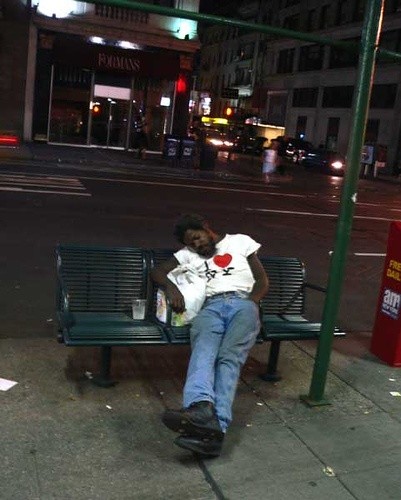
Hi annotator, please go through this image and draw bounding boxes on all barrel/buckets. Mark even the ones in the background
[155,286,189,326]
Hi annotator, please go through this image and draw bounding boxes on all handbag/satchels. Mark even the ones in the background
[157,262,207,326]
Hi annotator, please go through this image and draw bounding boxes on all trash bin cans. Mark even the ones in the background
[199,143,218,169]
[162,135,196,162]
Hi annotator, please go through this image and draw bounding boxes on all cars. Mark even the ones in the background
[195,127,346,174]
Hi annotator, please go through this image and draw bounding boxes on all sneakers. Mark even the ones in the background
[163,401,222,438]
[174,433,222,456]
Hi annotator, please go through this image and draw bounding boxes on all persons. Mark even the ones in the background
[262,143,277,184]
[151,213,269,458]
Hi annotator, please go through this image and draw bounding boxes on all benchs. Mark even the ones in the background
[47,245,346,388]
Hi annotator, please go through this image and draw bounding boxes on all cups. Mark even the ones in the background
[132,299,146,320]
